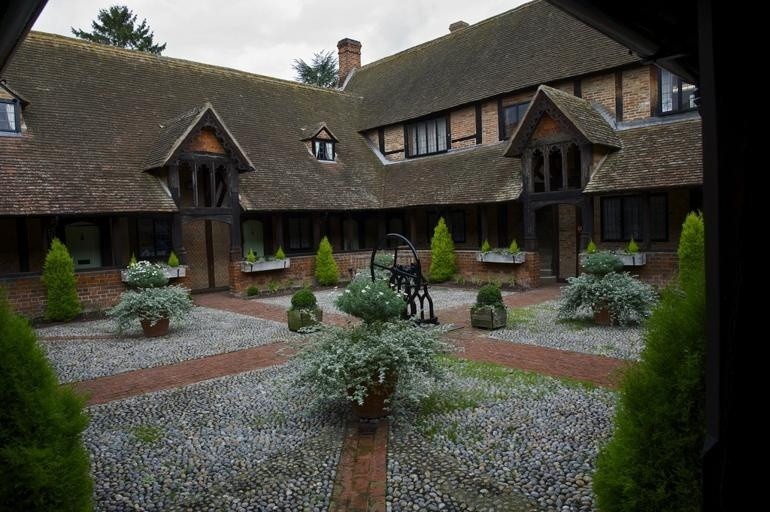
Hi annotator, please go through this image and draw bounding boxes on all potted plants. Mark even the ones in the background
[122,252,187,281]
[470,284,507,329]
[475,239,525,263]
[106,285,195,337]
[240,247,290,272]
[286,289,323,332]
[292,321,466,418]
[558,273,657,327]
[580,239,647,266]
